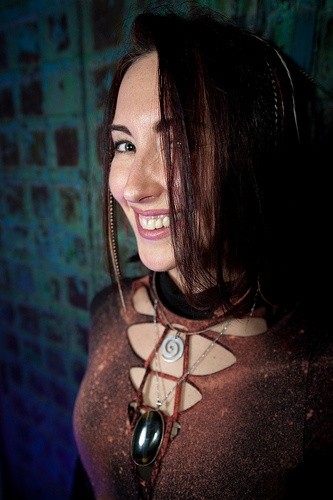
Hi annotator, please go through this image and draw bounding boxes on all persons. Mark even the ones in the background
[69,11,332,500]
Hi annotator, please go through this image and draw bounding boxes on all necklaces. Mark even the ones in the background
[129,301,237,468]
[148,271,259,365]
